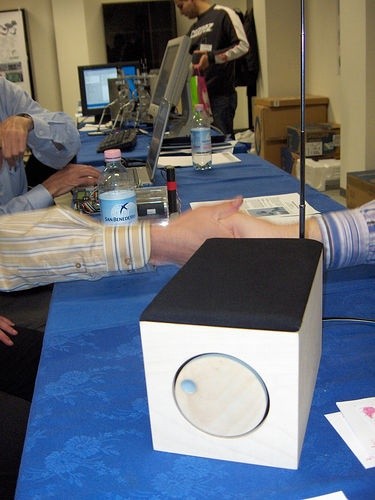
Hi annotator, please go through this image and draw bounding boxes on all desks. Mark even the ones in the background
[14,123,375,500]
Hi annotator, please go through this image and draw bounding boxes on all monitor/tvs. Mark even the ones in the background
[147,35,194,137]
[77,62,141,116]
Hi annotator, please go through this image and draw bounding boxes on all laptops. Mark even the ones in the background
[78,97,171,192]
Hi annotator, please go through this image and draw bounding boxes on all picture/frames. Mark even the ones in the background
[0,8,35,102]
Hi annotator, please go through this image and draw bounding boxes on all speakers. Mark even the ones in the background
[139,239,326,470]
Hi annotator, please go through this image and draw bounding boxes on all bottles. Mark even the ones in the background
[191,104,212,170]
[98,149,138,225]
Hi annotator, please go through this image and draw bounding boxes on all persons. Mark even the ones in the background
[0,196,244,483]
[0,77,101,333]
[220,199,375,271]
[174,0,250,140]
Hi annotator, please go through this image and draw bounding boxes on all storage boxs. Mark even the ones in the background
[138,234,325,471]
[285,124,344,160]
[253,94,329,107]
[295,160,347,210]
[252,103,327,167]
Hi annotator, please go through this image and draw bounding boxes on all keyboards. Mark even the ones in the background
[97,128,138,152]
